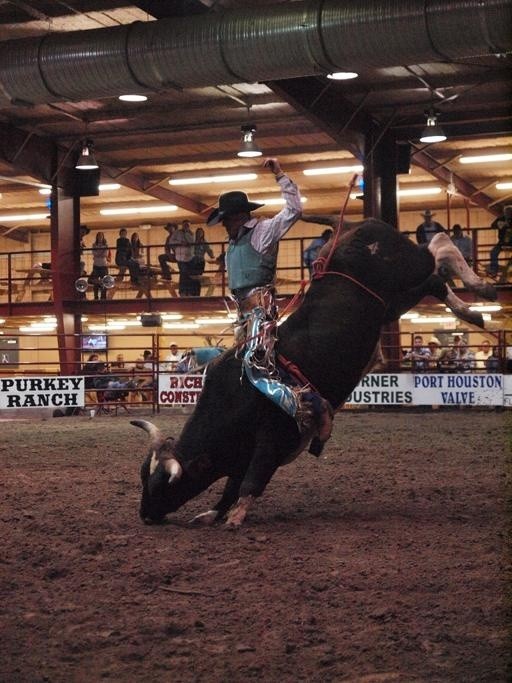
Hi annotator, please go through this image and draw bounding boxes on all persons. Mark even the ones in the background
[305,229,333,279]
[82,342,196,401]
[451,223,473,268]
[416,209,445,241]
[203,156,335,445]
[131,232,145,280]
[485,206,511,277]
[158,220,214,298]
[79,225,90,300]
[92,232,109,300]
[401,336,510,376]
[115,229,141,286]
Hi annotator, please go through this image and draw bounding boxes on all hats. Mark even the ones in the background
[421,210,436,217]
[207,191,265,227]
[428,337,441,346]
[164,223,178,230]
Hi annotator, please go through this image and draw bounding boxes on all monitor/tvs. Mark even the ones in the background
[81,332,107,352]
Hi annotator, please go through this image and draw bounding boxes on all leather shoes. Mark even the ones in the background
[318,397,332,441]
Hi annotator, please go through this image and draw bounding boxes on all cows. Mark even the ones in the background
[129,213,498,532]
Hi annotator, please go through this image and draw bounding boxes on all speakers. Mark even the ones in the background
[65,166,100,197]
[393,142,411,173]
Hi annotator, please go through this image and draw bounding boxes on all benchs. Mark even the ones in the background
[0,262,228,303]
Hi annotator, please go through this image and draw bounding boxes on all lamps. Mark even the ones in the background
[73,108,99,172]
[236,97,261,160]
[416,84,449,144]
